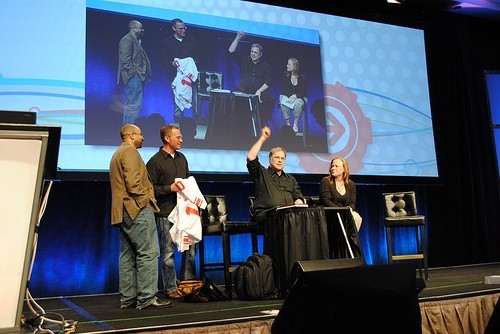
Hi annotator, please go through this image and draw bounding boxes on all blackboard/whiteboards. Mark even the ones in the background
[0,129,49,333]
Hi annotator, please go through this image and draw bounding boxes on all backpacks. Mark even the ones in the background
[233,253,275,301]
[178,116,197,148]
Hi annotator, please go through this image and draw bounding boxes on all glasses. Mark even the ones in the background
[129,132,142,136]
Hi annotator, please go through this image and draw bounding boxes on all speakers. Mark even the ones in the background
[286,258,363,297]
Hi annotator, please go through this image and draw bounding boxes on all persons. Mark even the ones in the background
[116,20,153,124]
[316,156,363,232]
[160,18,200,123]
[277,57,306,133]
[109,124,171,311]
[228,30,275,128]
[145,125,190,299]
[246,126,307,254]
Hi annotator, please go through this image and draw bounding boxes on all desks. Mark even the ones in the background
[204,89,262,145]
[266,207,362,299]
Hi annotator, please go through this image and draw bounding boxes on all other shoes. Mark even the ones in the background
[293,126,298,132]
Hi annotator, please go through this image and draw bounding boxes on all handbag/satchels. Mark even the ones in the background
[168,277,228,303]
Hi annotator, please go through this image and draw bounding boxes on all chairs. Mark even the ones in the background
[193,72,223,118]
[248,195,320,255]
[199,195,258,300]
[381,191,428,280]
[277,102,309,147]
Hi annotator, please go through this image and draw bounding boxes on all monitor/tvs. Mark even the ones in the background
[0,111,37,125]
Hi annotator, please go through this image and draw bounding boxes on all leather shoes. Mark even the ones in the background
[127,302,137,310]
[142,298,173,310]
